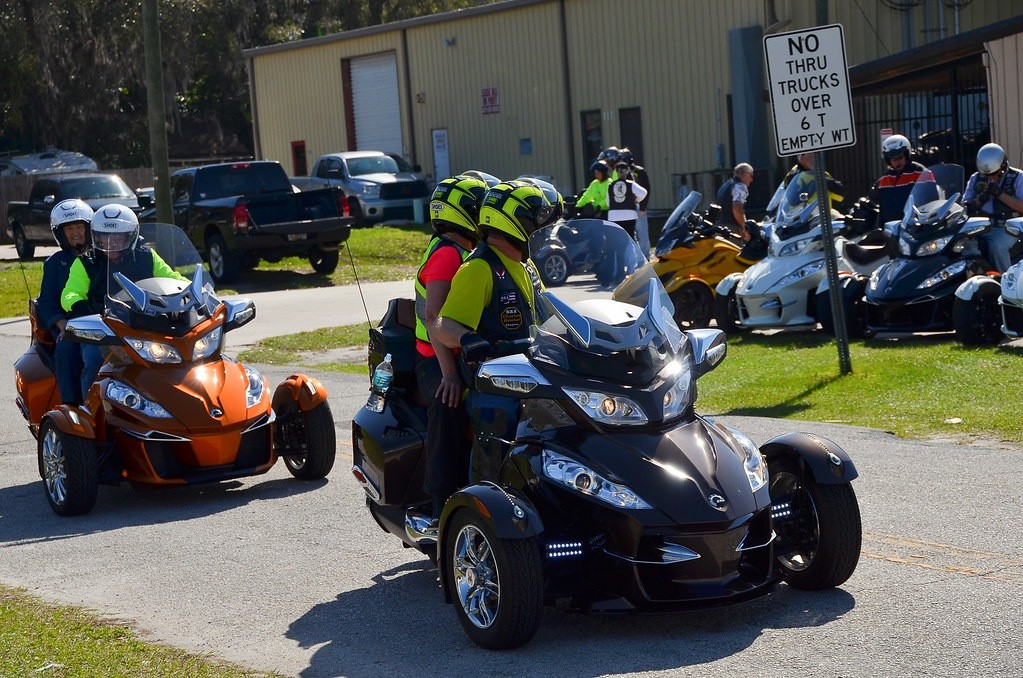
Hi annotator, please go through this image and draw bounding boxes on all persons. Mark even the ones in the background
[571,145,651,289]
[962,141,1023,274]
[873,134,940,231]
[409,166,566,524]
[714,161,753,243]
[783,151,844,211]
[35,199,207,414]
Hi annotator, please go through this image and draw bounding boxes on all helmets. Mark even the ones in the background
[92,204,139,258]
[597,147,620,164]
[49,199,94,252]
[590,160,612,183]
[431,170,502,250]
[479,177,564,260]
[976,144,1005,174]
[884,135,911,172]
[616,148,634,166]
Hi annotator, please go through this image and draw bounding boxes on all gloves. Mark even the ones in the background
[461,332,494,365]
[978,183,1002,202]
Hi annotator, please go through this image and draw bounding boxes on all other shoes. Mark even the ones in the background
[596,262,647,288]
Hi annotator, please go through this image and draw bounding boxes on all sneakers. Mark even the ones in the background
[404,506,440,544]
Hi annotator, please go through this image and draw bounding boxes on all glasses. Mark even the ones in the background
[892,156,905,163]
[985,170,999,176]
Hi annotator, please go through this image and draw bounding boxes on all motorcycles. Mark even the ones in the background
[527,187,609,287]
[610,164,1023,348]
[352,214,863,654]
[15,220,335,517]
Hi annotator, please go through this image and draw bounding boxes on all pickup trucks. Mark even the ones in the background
[289,149,433,229]
[6,170,146,261]
[137,161,353,286]
